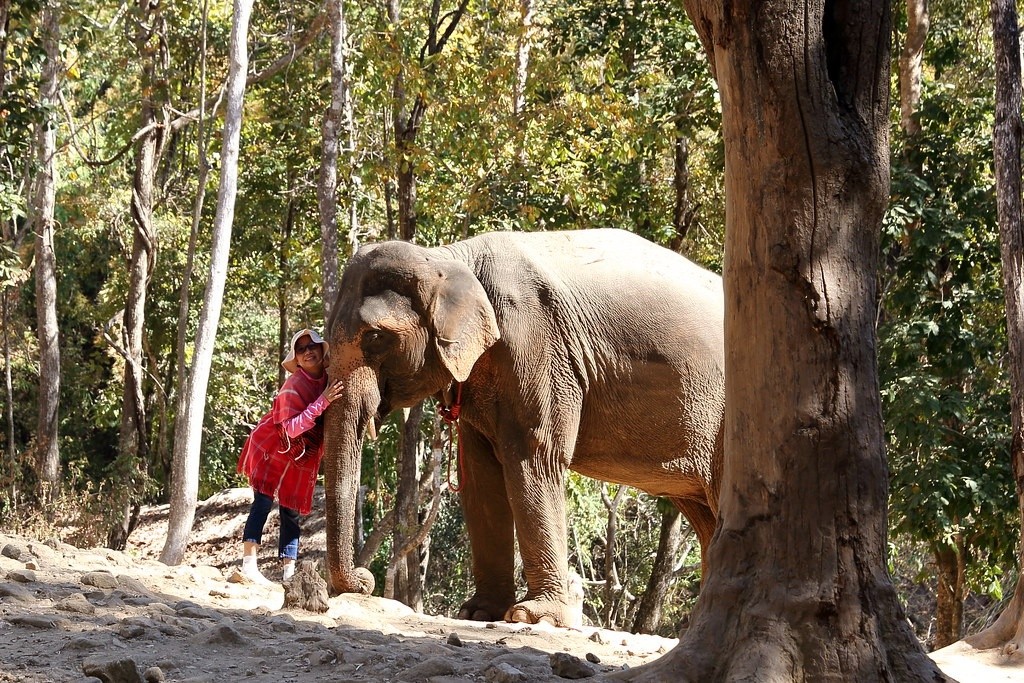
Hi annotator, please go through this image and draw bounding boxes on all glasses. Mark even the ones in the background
[295,340,318,354]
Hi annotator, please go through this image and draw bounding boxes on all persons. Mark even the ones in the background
[235,329,344,584]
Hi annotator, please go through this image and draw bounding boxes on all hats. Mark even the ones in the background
[281,328,331,373]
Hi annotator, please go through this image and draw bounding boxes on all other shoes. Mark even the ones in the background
[241,567,274,587]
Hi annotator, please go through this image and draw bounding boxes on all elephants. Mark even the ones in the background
[322,228,726,635]
